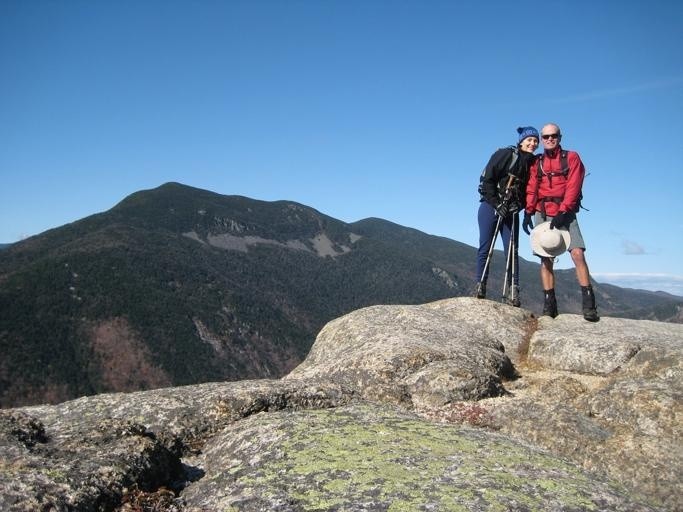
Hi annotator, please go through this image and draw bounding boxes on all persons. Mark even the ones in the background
[478,127,543,307]
[523,124,597,322]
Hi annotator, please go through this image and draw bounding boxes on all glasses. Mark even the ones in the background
[542,134,559,140]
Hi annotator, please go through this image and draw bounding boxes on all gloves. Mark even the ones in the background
[509,201,522,214]
[496,203,509,218]
[549,211,574,230]
[522,211,534,236]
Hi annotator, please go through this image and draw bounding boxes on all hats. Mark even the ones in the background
[528,221,571,259]
[516,126,540,146]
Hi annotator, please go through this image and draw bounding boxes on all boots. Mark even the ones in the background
[538,287,560,319]
[475,281,487,299]
[507,285,521,308]
[580,283,600,322]
